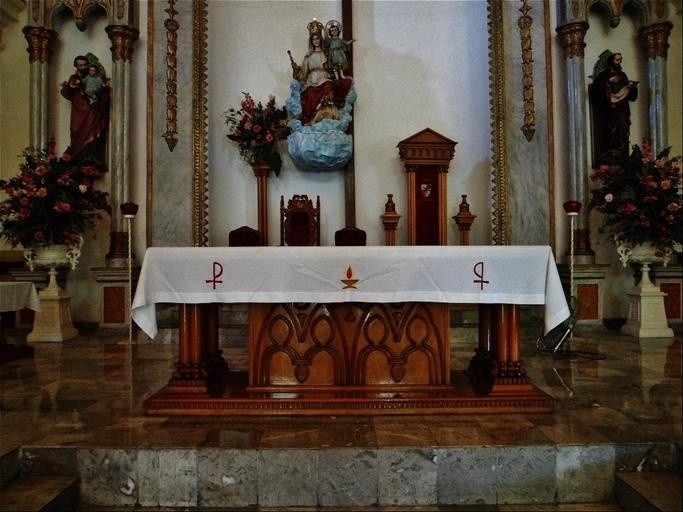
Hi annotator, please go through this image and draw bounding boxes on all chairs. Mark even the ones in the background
[281,195,320,246]
[334,225,366,246]
[229,226,261,246]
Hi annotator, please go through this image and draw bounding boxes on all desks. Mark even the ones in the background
[132,246,569,413]
[1,275,43,328]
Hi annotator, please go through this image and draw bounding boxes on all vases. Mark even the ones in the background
[616,238,673,267]
[24,243,78,268]
[254,152,265,161]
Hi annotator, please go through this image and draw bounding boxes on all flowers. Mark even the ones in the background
[223,91,291,178]
[587,137,683,245]
[0,132,112,245]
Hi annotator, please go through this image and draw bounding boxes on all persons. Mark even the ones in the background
[592,51,638,159]
[75,65,106,105]
[322,18,358,79]
[61,54,109,168]
[289,34,337,129]
